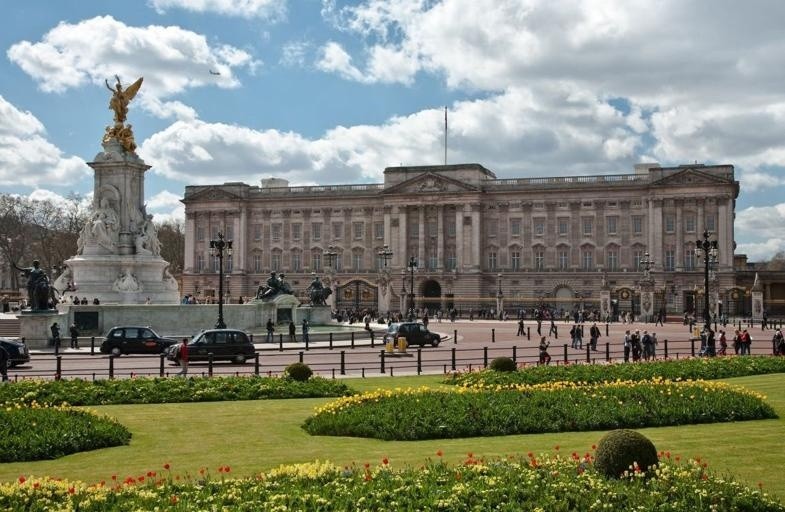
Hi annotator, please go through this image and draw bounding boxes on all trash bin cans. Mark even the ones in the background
[386,337,394,353]
[398,337,406,352]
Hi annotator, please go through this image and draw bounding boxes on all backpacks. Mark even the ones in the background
[166,342,184,361]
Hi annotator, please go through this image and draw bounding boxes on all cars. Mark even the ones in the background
[380,321,442,348]
[164,328,256,367]
[97,323,177,358]
[1,336,34,377]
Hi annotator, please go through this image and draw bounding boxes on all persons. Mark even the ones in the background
[181,294,214,304]
[516,316,526,336]
[539,336,552,366]
[0,294,8,312]
[105,78,130,126]
[116,123,137,152]
[301,319,310,342]
[10,257,46,309]
[17,299,27,310]
[289,320,298,342]
[69,323,81,350]
[682,309,785,356]
[175,337,190,378]
[277,273,294,294]
[243,295,249,304]
[203,334,213,344]
[264,319,274,343]
[623,329,659,364]
[655,308,664,327]
[88,195,120,254]
[144,296,152,305]
[238,296,243,304]
[536,317,543,336]
[476,305,635,324]
[50,322,61,348]
[548,319,559,337]
[570,325,578,348]
[56,280,100,305]
[306,275,324,304]
[330,307,476,338]
[89,212,114,238]
[143,214,161,257]
[10,303,19,313]
[574,324,585,350]
[254,270,281,299]
[590,322,602,351]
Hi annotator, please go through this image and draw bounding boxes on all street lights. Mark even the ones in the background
[206,231,235,329]
[497,271,503,296]
[378,242,395,267]
[694,227,721,357]
[406,255,419,316]
[323,243,338,267]
[47,265,57,283]
[639,252,656,282]
[310,270,316,281]
[701,254,720,273]
[223,272,232,304]
